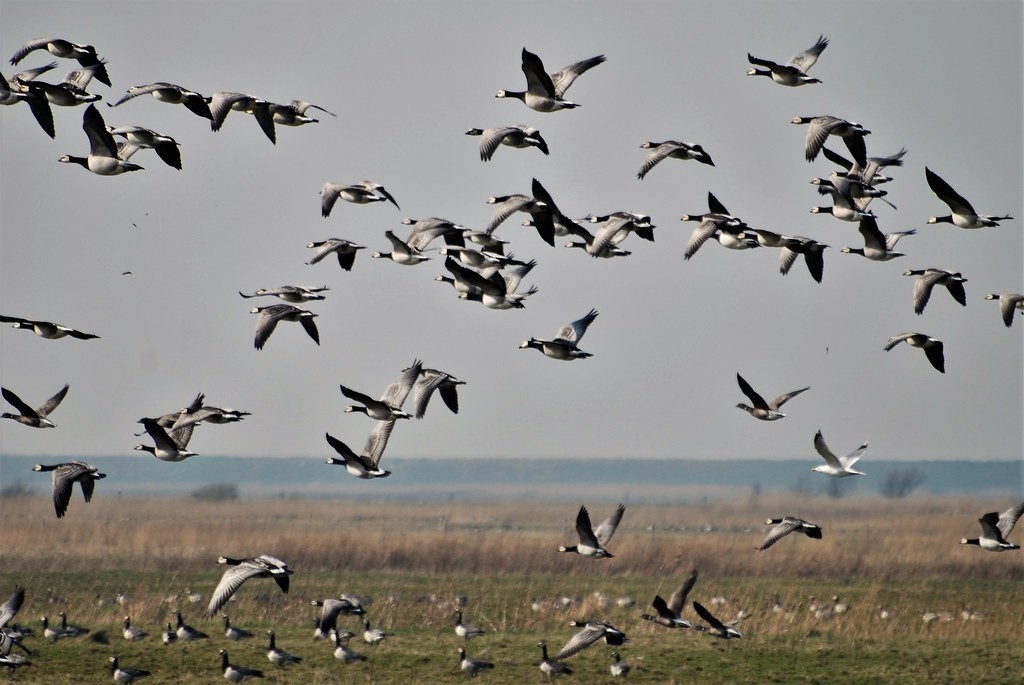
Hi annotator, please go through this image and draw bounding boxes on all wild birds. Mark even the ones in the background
[639,570,697,628]
[692,600,752,638]
[553,620,629,662]
[810,429,869,477]
[752,517,822,551]
[958,502,1024,551]
[455,608,486,640]
[537,642,574,684]
[528,593,987,628]
[132,393,252,462]
[0,315,101,340]
[555,504,627,559]
[0,33,1024,437]
[1,385,69,428]
[735,373,811,421]
[0,556,395,685]
[609,653,628,677]
[32,462,107,519]
[457,648,496,677]
[325,419,394,478]
